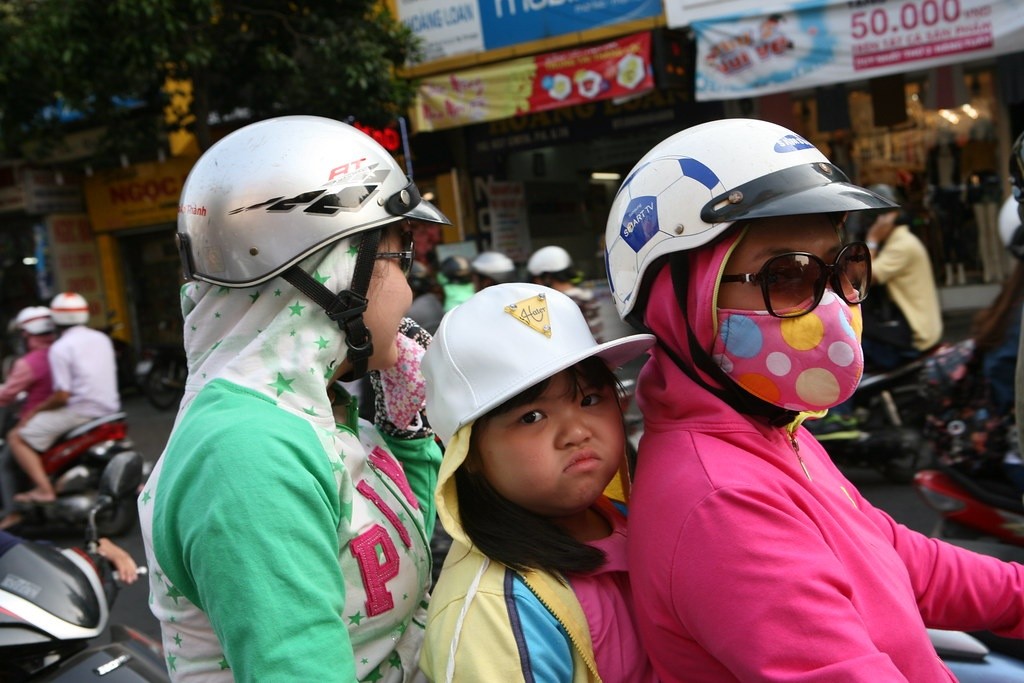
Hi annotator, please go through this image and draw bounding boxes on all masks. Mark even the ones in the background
[711,288,864,410]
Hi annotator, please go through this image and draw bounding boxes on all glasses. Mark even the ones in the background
[720,242,872,318]
[374,236,416,279]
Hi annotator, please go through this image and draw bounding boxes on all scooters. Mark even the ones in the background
[800,339,941,486]
[0,355,154,541]
[0,448,175,682]
[132,314,188,413]
[909,443,1024,566]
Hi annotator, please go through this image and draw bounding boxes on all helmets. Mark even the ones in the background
[174,115,453,289]
[15,305,55,333]
[997,194,1021,248]
[471,252,514,276]
[603,119,900,325]
[50,293,89,326]
[526,246,572,275]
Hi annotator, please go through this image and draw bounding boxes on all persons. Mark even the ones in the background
[139,115,452,683]
[420,282,656,683]
[925,123,969,286]
[406,260,442,333]
[0,529,138,585]
[471,250,515,291]
[435,254,472,312]
[863,183,944,373]
[0,291,121,530]
[976,196,1024,417]
[528,247,605,344]
[603,119,1024,683]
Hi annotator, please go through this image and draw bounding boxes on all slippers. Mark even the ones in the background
[13,493,56,504]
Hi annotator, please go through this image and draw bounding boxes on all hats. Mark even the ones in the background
[420,282,657,446]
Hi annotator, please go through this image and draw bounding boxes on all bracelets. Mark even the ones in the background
[866,242,877,249]
